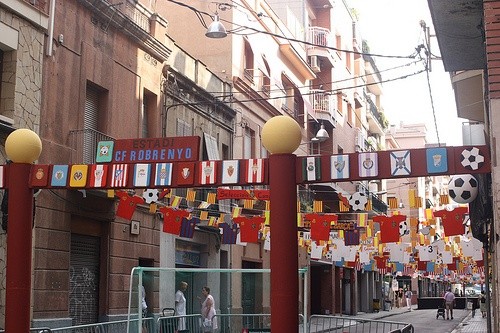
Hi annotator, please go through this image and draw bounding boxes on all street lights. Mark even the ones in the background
[3,128,43,333]
[260,115,303,333]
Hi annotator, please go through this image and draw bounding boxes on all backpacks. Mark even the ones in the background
[480,295,486,304]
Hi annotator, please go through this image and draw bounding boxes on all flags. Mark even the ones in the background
[106,188,486,284]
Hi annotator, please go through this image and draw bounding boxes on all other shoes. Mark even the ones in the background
[447,318,449,320]
[451,317,453,320]
[484,314,486,316]
[482,316,484,318]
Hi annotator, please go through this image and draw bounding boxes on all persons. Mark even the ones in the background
[397,286,412,312]
[138,281,156,333]
[197,285,218,333]
[443,286,456,321]
[173,281,190,333]
[477,290,489,320]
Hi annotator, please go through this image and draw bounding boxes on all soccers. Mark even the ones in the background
[448,175,479,204]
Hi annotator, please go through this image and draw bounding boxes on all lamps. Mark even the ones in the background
[316,121,329,140]
[205,13,227,38]
